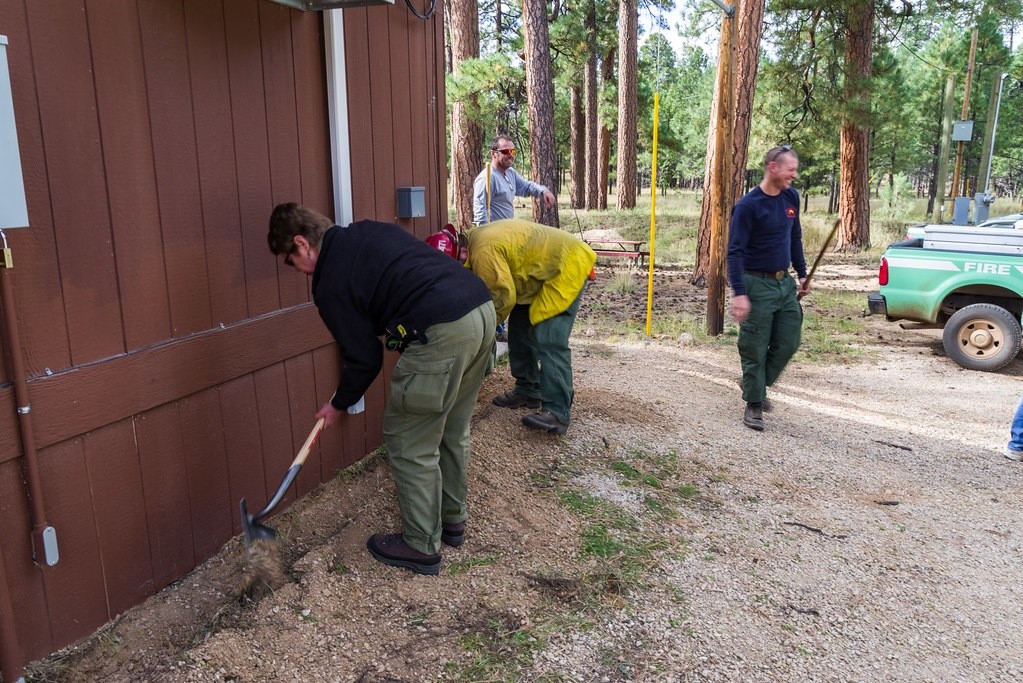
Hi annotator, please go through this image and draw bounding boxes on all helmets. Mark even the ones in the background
[423,223,459,261]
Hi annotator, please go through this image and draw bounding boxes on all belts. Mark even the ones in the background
[756,270,788,281]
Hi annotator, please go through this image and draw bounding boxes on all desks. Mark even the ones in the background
[583,239,647,268]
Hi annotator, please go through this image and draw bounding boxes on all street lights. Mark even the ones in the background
[983,71,1010,198]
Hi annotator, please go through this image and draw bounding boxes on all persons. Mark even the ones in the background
[267,199,498,576]
[726,146,813,432]
[1003,396,1023,461]
[472,134,555,343]
[425,218,598,436]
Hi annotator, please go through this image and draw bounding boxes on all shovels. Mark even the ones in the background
[240,389,338,549]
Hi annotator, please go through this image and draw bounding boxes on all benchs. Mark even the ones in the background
[592,248,650,267]
[594,250,641,271]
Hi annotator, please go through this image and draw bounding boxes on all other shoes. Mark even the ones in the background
[1003,447,1023,461]
[493,388,541,410]
[738,380,770,412]
[495,330,508,342]
[367,533,441,575]
[440,521,466,547]
[742,403,764,431]
[522,410,568,435]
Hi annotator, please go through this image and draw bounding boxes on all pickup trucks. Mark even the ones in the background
[904,209,1023,242]
[867,231,1023,371]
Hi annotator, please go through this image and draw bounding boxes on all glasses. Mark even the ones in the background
[281,230,309,267]
[493,149,517,156]
[771,145,791,162]
